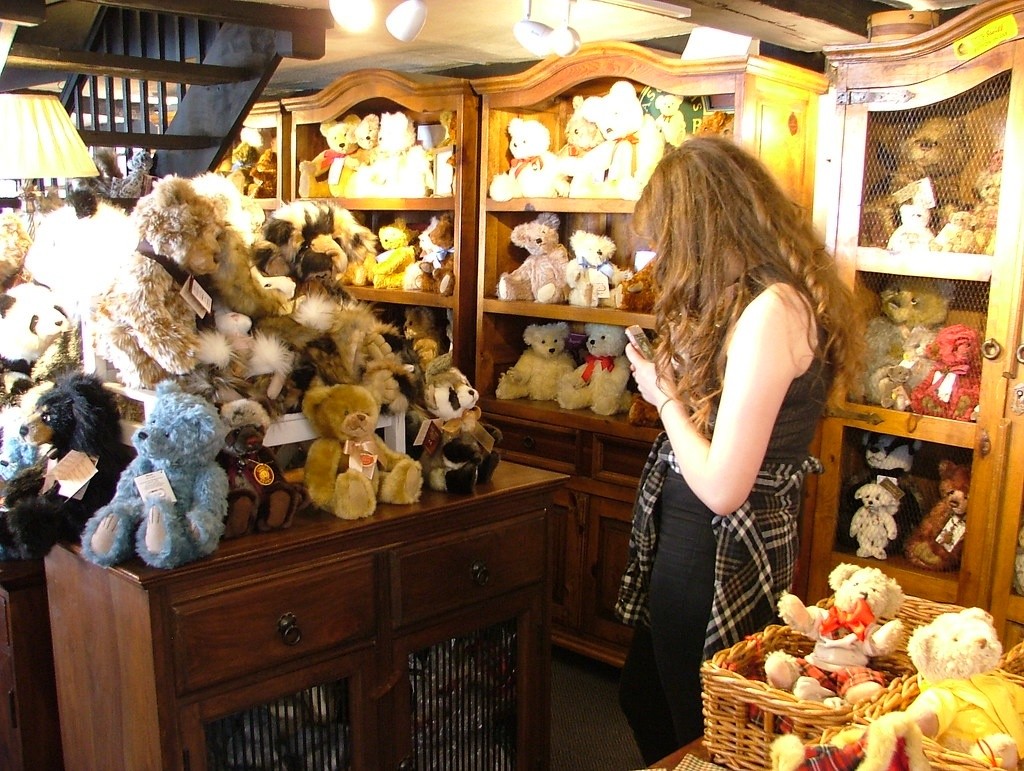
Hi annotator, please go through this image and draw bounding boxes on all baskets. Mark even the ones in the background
[702,593,974,771]
[922,644,1024,771]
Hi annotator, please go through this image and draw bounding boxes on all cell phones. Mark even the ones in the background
[626,324,656,363]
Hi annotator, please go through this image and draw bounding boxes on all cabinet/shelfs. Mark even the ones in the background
[0,68,1024,771]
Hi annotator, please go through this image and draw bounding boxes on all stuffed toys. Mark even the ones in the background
[81,380,228,570]
[614,238,666,314]
[845,275,980,423]
[495,212,571,304]
[298,110,457,198]
[489,119,571,203]
[557,323,632,416]
[557,80,734,200]
[764,563,905,707]
[864,116,1006,253]
[566,230,633,307]
[843,431,971,571]
[771,712,931,771]
[906,607,1024,771]
[496,323,576,401]
[0,127,504,560]
[302,384,423,520]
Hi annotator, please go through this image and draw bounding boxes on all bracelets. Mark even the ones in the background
[659,399,672,418]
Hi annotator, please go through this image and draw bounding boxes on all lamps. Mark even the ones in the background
[514,0,580,57]
[0,94,99,244]
[385,0,427,41]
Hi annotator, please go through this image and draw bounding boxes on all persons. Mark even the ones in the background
[621,136,865,768]
